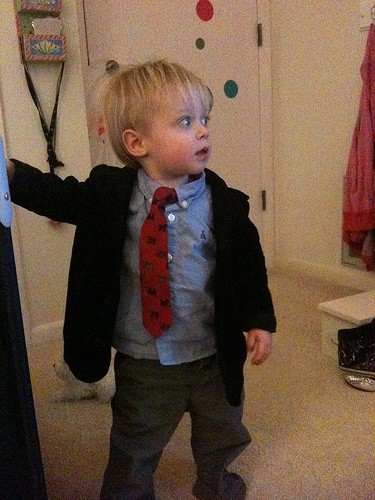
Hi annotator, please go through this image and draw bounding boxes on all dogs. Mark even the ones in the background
[54,346,115,405]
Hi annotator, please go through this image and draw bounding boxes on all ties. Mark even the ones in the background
[137,187,179,336]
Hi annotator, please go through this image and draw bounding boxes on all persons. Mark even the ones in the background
[5,59,276,500]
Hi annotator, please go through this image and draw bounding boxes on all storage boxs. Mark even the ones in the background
[318,289,375,361]
